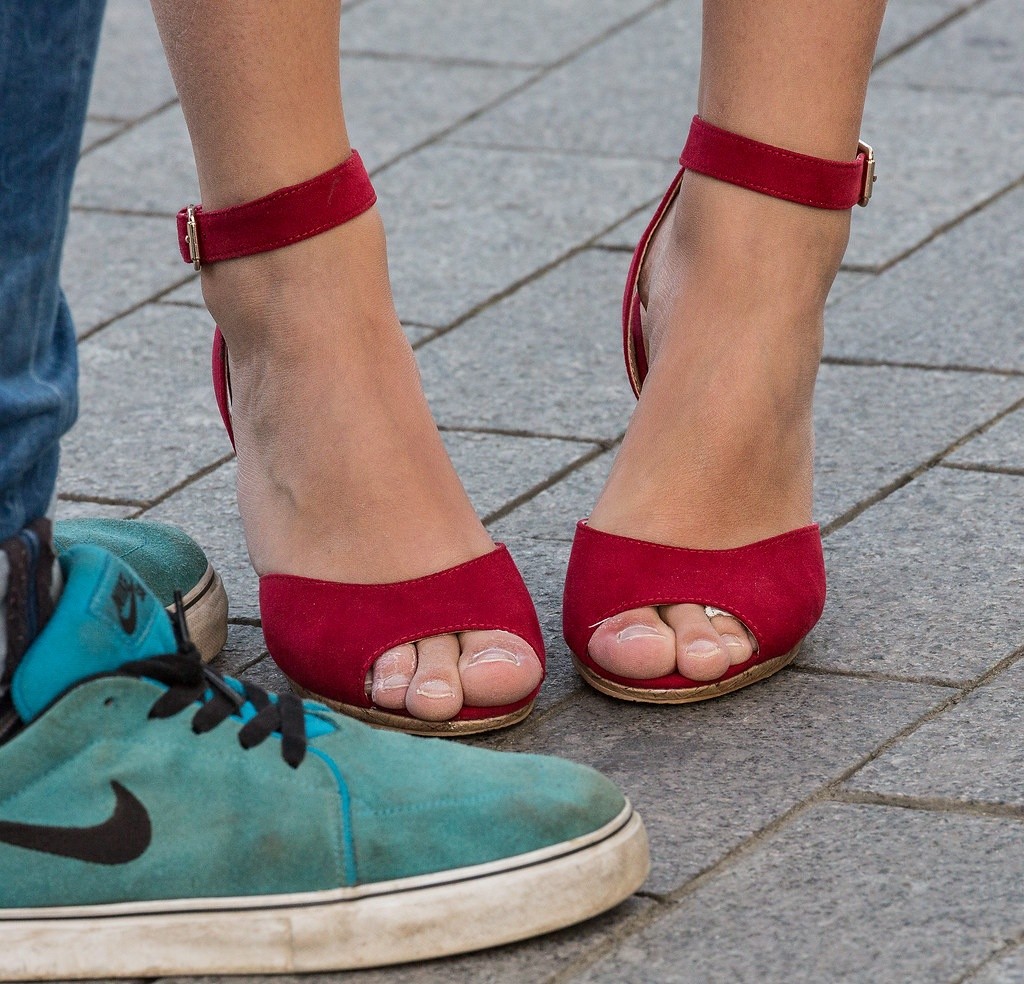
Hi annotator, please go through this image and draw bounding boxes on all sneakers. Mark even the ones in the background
[45,520,230,667]
[0,542,650,980]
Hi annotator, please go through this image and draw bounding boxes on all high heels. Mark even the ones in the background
[177,147,543,735]
[559,113,875,705]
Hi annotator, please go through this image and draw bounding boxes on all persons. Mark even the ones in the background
[0,0,893,974]
